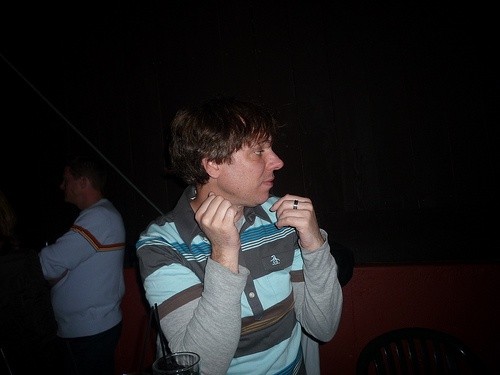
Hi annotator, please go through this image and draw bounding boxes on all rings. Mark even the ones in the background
[293,199,298,210]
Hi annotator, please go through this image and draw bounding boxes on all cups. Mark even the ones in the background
[153,352,201,375]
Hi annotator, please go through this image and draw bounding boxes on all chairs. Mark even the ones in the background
[356,327,482,375]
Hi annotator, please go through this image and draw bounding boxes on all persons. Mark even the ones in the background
[135,80,343,375]
[38,160,125,375]
